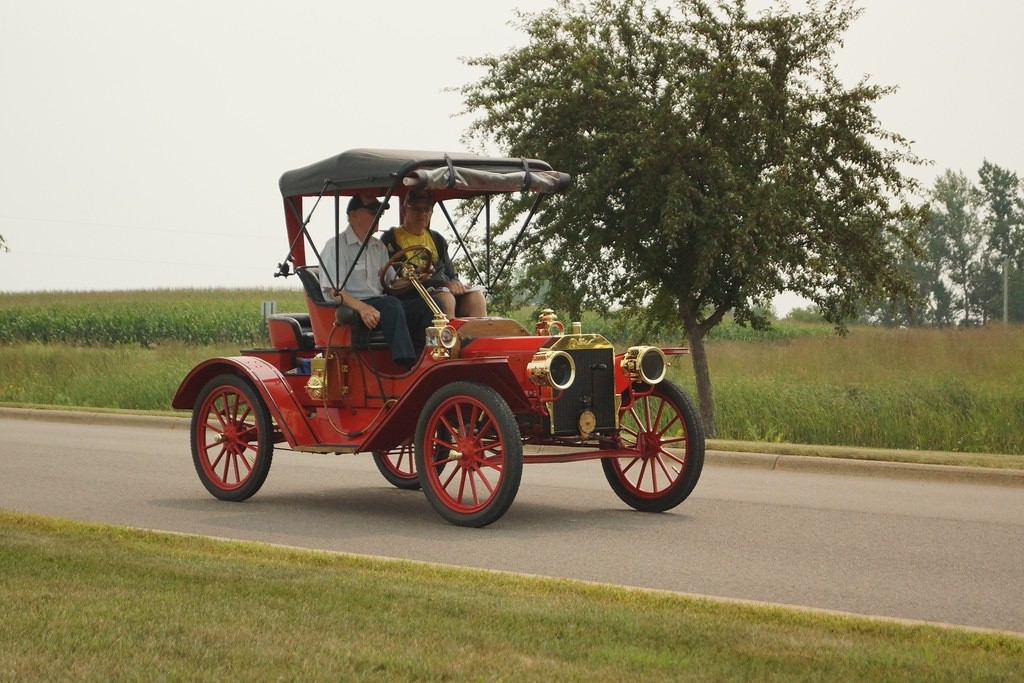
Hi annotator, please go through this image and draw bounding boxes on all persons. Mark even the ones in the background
[380,189,486,321]
[319,195,447,371]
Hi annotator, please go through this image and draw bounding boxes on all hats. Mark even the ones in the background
[404,189,436,207]
[346,193,390,212]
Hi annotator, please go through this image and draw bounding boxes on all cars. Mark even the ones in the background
[171,148,707,530]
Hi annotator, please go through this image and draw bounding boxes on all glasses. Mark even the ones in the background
[361,208,384,215]
[407,205,433,214]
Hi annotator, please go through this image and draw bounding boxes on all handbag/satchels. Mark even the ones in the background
[424,260,449,286]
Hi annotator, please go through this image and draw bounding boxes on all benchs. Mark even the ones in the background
[297,265,387,347]
[267,313,314,348]
